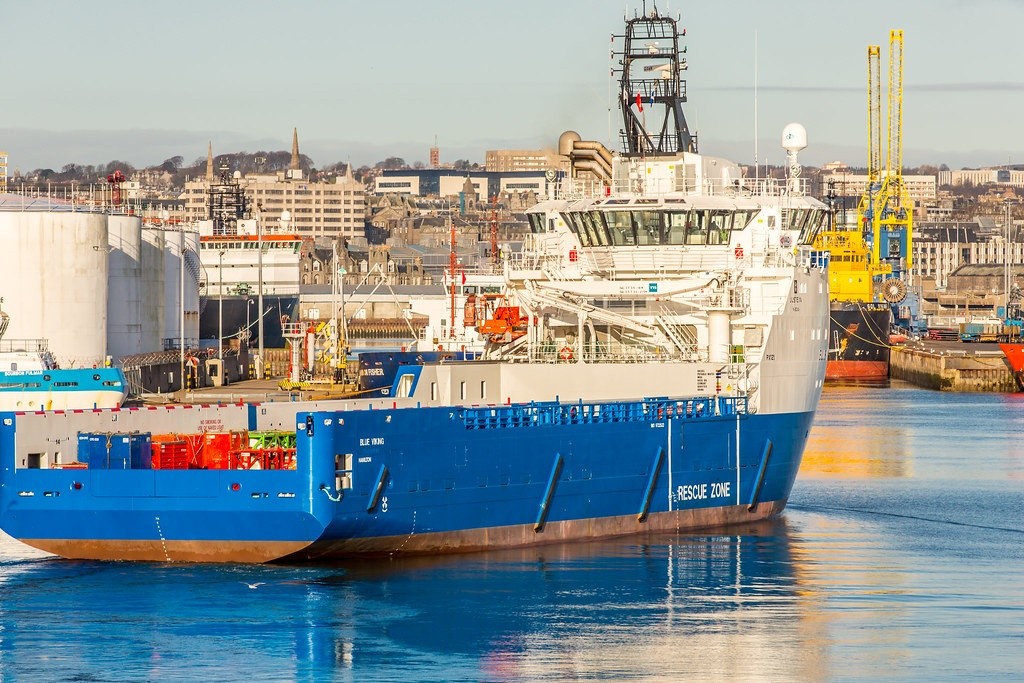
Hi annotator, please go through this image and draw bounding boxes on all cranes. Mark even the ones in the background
[854,29,914,279]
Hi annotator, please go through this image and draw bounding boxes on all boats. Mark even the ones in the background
[803,179,894,387]
[0,0,833,567]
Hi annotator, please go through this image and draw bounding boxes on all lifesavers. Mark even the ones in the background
[561,348,573,360]
